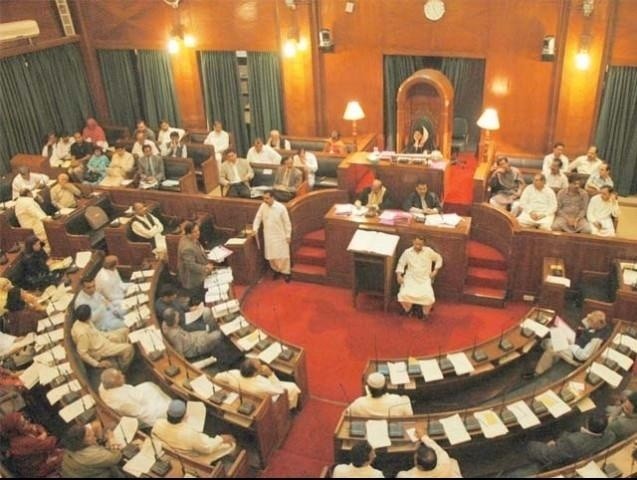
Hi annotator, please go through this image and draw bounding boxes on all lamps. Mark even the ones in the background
[168,25,184,54]
[343,101,365,137]
[281,28,299,59]
[573,34,593,72]
[475,108,501,142]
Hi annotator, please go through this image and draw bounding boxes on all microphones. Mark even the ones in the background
[386,406,406,436]
[437,301,548,371]
[162,339,180,378]
[134,248,164,362]
[557,318,637,402]
[236,382,256,415]
[176,455,199,478]
[462,382,548,429]
[348,398,367,438]
[118,421,140,459]
[427,404,444,436]
[180,339,195,390]
[208,372,228,405]
[407,349,420,373]
[142,429,171,477]
[538,439,637,480]
[41,248,113,443]
[254,319,272,350]
[374,346,390,376]
[276,325,294,360]
[212,261,251,338]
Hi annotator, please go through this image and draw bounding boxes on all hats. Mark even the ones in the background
[367,371,385,390]
[166,399,186,420]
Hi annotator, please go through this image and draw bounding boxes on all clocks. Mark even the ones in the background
[424,0,445,20]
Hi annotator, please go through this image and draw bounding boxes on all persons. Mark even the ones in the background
[345,373,413,415]
[1,114,316,480]
[355,179,443,215]
[330,429,463,479]
[324,129,351,155]
[394,234,444,322]
[520,310,610,378]
[402,121,437,153]
[519,389,637,465]
[487,143,621,237]
